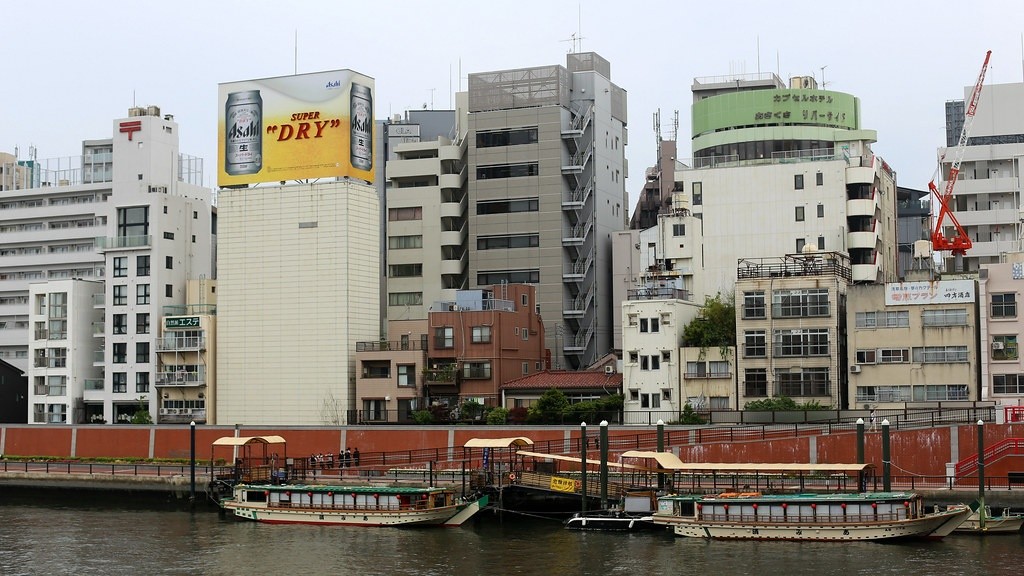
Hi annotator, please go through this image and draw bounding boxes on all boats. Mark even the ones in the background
[204,434,533,529]
[565,448,980,542]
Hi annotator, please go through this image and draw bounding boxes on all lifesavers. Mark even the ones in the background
[509,474,515,479]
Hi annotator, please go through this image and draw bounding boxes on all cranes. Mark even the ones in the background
[928,49,993,273]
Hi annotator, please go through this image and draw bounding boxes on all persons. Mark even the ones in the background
[317,452,324,467]
[352,447,360,466]
[324,452,335,469]
[344,448,351,467]
[867,406,877,431]
[309,453,317,468]
[338,450,345,468]
